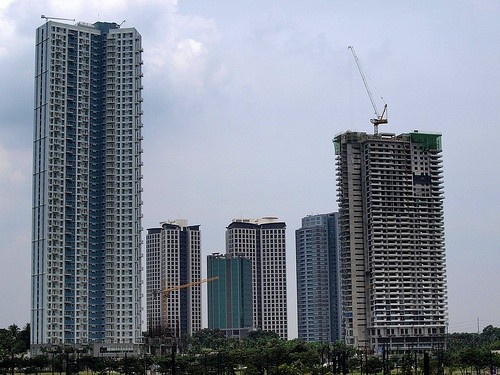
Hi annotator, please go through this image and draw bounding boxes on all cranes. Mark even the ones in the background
[348,46,388,134]
[41,14,75,24]
[153,276,217,339]
[116,20,126,28]
